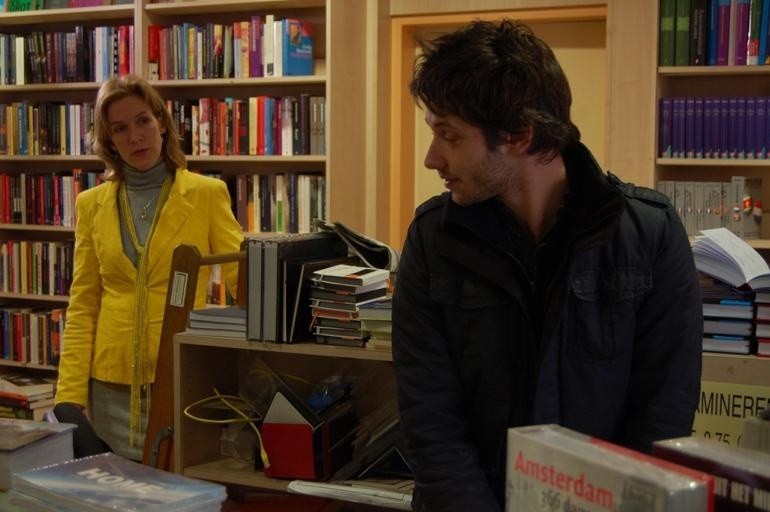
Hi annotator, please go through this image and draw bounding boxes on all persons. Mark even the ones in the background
[392,18,702,509]
[53,75,246,466]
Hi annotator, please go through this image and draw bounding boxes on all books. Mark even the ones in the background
[185,217,394,350]
[2,169,104,227]
[190,170,326,234]
[657,0,768,353]
[2,417,227,510]
[149,15,315,79]
[2,306,66,367]
[505,421,715,511]
[3,99,99,156]
[2,239,75,296]
[2,373,58,421]
[2,25,134,85]
[6,2,111,12]
[289,408,415,507]
[653,434,770,509]
[163,94,326,157]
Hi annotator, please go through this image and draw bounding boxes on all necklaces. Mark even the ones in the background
[131,192,157,220]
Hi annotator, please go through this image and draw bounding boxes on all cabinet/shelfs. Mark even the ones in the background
[643,0,769,442]
[0,1,368,434]
[171,328,414,511]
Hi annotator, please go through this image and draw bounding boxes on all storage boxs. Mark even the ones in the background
[257,400,357,483]
